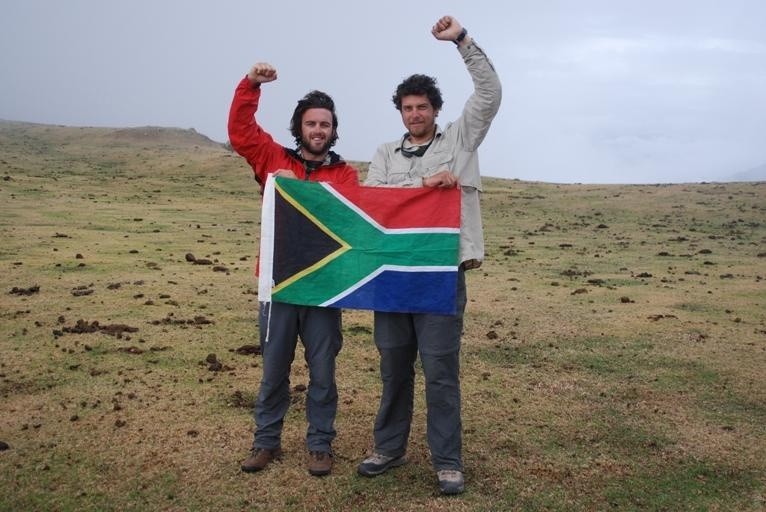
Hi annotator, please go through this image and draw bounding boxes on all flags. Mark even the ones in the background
[256,171,462,317]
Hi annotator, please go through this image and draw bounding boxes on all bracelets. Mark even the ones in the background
[453,25,468,46]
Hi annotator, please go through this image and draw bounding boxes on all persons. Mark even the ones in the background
[353,13,504,497]
[224,59,362,479]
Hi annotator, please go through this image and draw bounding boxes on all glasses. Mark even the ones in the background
[400,139,433,158]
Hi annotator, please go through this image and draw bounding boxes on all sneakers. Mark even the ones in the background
[240,447,281,472]
[307,451,331,476]
[436,470,465,494]
[357,452,408,477]
